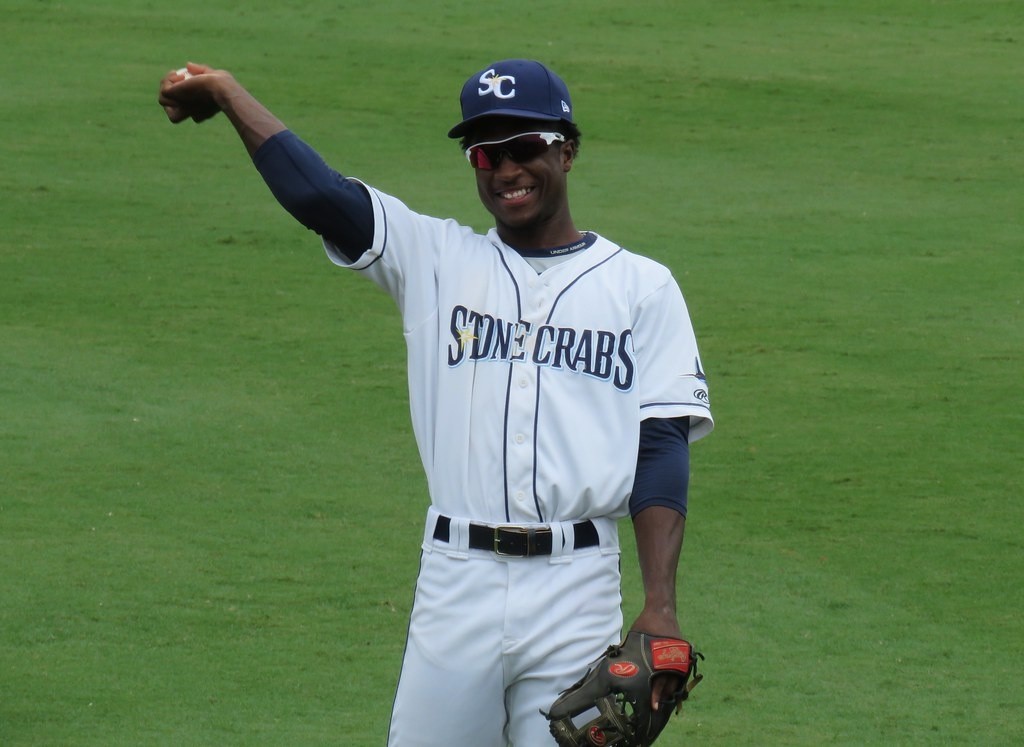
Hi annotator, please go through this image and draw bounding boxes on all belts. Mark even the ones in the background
[432,515,599,558]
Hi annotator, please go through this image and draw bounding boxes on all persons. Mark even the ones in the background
[159,60,714,747]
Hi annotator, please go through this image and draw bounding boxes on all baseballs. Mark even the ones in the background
[176,67,194,80]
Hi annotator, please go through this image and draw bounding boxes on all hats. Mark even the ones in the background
[447,59,574,139]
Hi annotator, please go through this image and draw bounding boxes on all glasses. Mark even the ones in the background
[464,132,564,171]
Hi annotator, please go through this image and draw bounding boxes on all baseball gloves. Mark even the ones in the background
[539,626,705,747]
